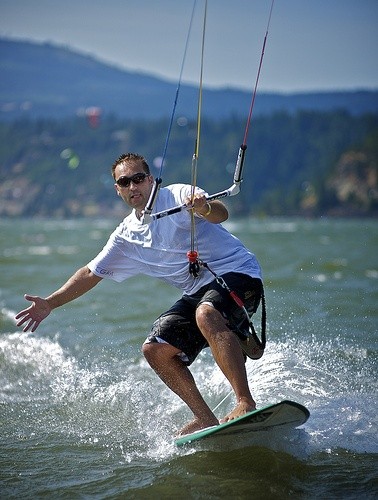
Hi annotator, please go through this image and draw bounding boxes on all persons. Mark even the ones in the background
[15,152,266,435]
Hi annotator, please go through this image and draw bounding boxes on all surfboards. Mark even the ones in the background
[175,400,311,449]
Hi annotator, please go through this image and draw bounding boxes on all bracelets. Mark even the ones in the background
[196,202,211,218]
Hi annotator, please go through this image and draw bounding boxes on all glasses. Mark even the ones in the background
[116,172,148,188]
